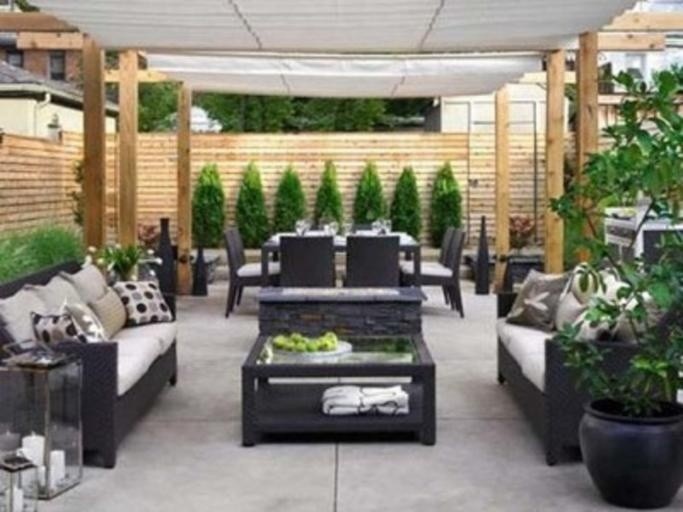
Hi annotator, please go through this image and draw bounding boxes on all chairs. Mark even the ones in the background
[222,228,280,317]
[345,236,399,286]
[403,229,467,318]
[280,235,335,286]
[399,228,455,310]
[231,226,280,305]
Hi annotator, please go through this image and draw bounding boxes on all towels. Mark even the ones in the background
[323,384,411,416]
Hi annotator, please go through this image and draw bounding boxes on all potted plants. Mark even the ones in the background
[543,61,683,509]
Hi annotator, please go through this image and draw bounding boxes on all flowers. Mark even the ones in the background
[81,243,164,282]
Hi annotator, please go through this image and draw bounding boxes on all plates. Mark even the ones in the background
[263,340,353,359]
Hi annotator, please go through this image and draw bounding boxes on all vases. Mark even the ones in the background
[476,216,490,295]
[155,218,177,320]
[191,244,207,296]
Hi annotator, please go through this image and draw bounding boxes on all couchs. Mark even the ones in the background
[496,264,677,465]
[0,255,179,467]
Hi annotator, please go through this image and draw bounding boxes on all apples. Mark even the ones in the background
[272,333,338,353]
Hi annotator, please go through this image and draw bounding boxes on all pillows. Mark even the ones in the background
[505,268,662,345]
[1,265,174,353]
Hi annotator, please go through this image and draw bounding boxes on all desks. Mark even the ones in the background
[255,283,427,334]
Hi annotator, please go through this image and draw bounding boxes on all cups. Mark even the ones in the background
[295,220,306,237]
[343,218,354,237]
[372,216,393,236]
